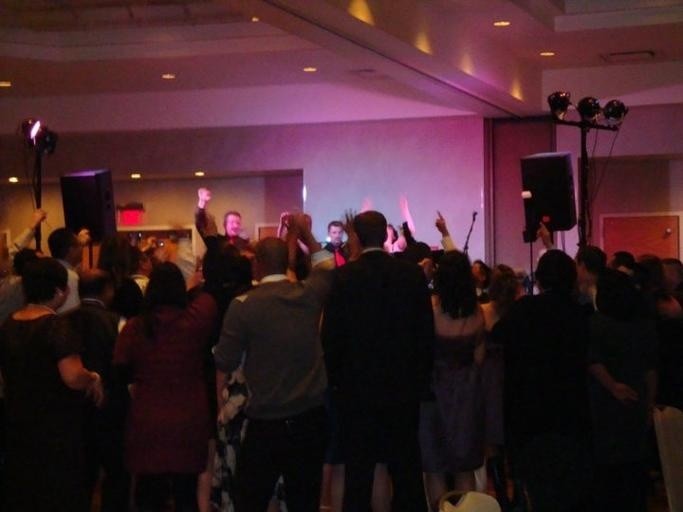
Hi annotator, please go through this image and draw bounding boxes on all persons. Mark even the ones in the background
[0,186,683,511]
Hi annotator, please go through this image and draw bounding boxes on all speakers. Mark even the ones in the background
[521,151,577,231]
[60,169,116,245]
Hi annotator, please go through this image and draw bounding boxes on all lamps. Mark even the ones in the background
[532,89,630,252]
[13,114,57,248]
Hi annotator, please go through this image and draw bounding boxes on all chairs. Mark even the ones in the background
[436,486,507,511]
[645,402,682,510]
